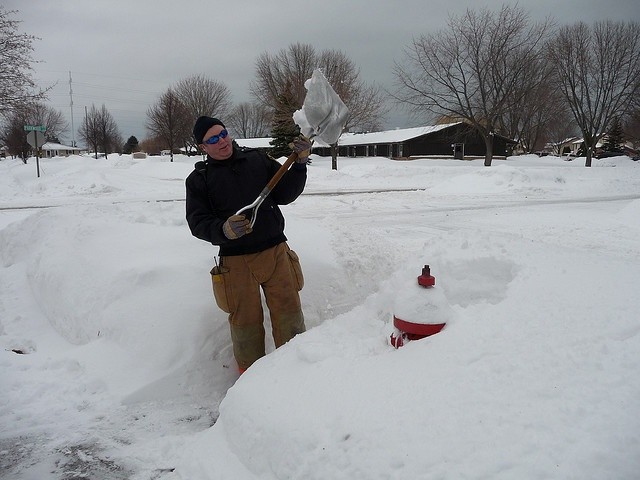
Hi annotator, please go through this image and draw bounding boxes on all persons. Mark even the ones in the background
[184,116,308,376]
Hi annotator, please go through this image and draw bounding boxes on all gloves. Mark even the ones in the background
[219,215,254,240]
[288,133,312,163]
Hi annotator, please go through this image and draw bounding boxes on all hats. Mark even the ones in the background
[193,115,225,144]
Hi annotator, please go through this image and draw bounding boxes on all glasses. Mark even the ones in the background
[200,128,228,144]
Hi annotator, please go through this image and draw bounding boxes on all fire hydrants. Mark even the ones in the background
[391,265,446,348]
